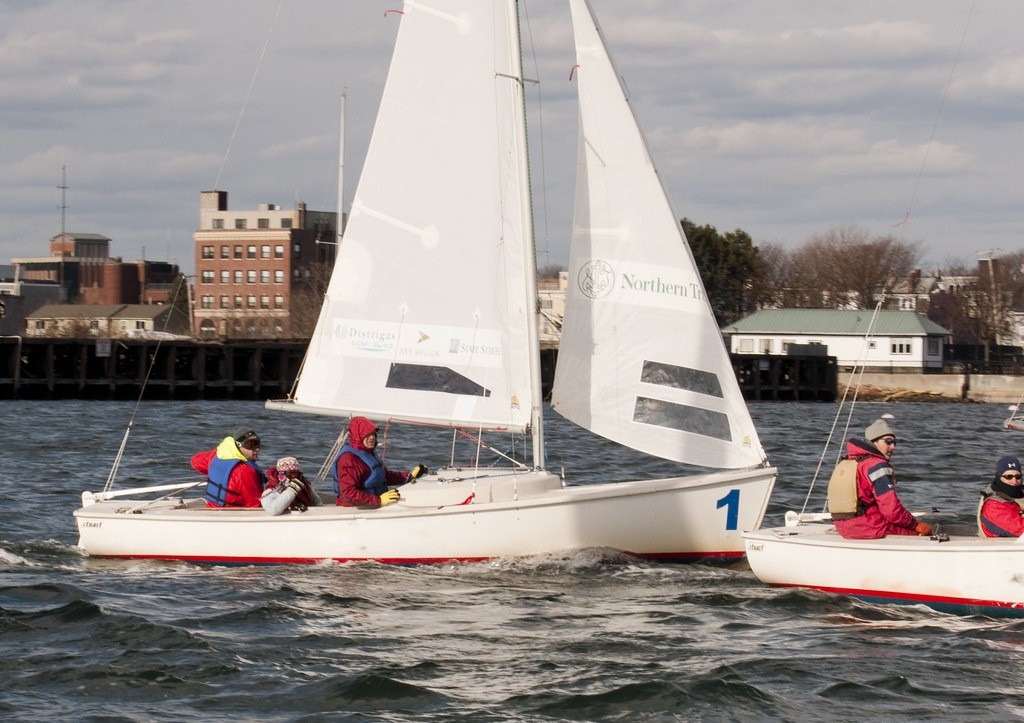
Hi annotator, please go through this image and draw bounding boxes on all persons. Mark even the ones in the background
[832,419,933,541]
[259,457,322,516]
[975,457,1024,539]
[189,426,265,508]
[334,416,428,510]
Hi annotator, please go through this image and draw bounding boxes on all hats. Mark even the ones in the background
[864,419,896,442]
[995,456,1022,480]
[276,456,301,471]
[232,425,255,441]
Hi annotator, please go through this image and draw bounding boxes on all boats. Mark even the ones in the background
[743,519,1024,618]
[1004,415,1024,431]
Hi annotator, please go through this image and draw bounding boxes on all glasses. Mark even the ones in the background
[242,438,260,450]
[1001,473,1022,480]
[881,438,897,446]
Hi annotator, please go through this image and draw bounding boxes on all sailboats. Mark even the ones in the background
[72,0,776,563]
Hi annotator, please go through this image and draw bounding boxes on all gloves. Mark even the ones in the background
[916,522,932,536]
[379,488,401,506]
[289,478,305,495]
[410,463,429,479]
